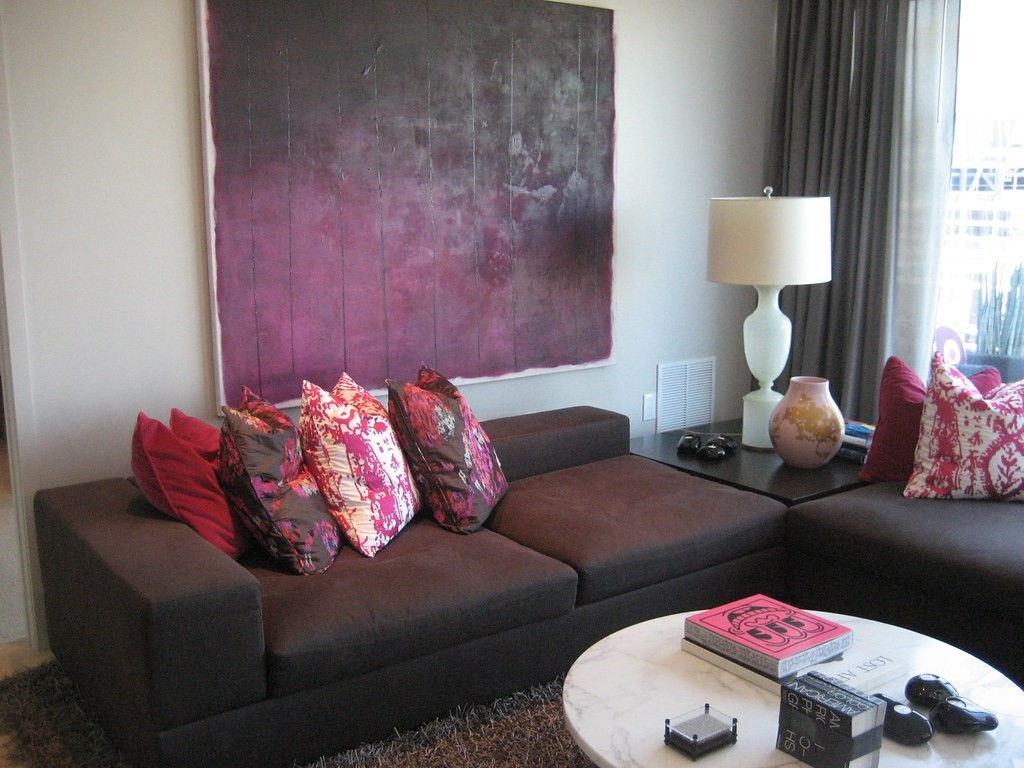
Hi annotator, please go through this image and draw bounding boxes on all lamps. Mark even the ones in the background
[707,196,831,453]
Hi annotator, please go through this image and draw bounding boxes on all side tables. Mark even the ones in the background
[630,418,874,507]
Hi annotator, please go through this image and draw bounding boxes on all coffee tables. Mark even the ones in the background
[562,609,1024,768]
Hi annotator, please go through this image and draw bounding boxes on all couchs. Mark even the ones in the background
[789,354,1024,688]
[32,406,789,768]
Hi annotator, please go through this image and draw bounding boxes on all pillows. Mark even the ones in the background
[384,361,510,534]
[298,372,422,556]
[217,384,339,574]
[856,355,1001,482]
[904,351,1024,502]
[131,408,256,562]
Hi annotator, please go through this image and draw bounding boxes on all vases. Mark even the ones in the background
[768,377,846,467]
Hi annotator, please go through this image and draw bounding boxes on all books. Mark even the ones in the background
[776,672,886,768]
[681,637,910,703]
